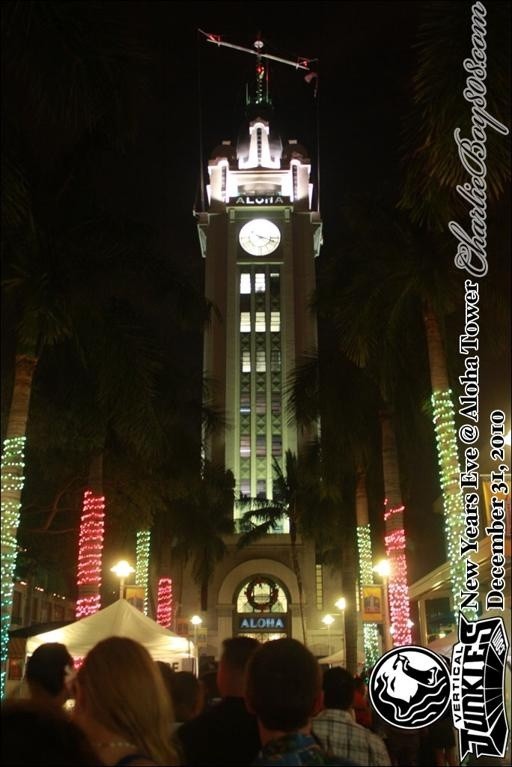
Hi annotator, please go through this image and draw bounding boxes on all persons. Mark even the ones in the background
[0,630,457,766]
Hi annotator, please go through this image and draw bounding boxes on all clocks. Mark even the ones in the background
[238,218,281,258]
